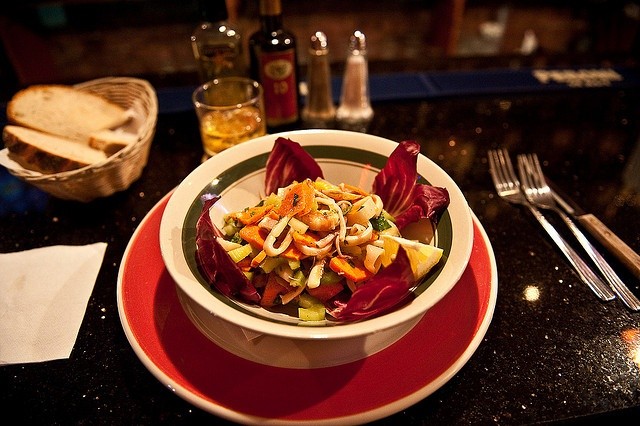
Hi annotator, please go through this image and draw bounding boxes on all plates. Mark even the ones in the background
[116,186,500,426]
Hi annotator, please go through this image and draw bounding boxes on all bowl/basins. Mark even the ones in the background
[157,127,475,342]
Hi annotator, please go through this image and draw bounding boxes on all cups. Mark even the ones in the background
[191,76,266,156]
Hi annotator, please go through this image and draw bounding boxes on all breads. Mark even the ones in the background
[6,82,129,138]
[89,130,133,153]
[3,125,104,173]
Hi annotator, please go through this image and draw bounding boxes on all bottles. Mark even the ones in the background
[336,29,376,123]
[190,0,244,81]
[247,0,301,129]
[300,30,336,123]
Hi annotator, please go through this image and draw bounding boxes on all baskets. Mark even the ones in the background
[6,76,158,202]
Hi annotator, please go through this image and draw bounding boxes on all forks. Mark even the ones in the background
[516,152,640,311]
[486,147,616,302]
